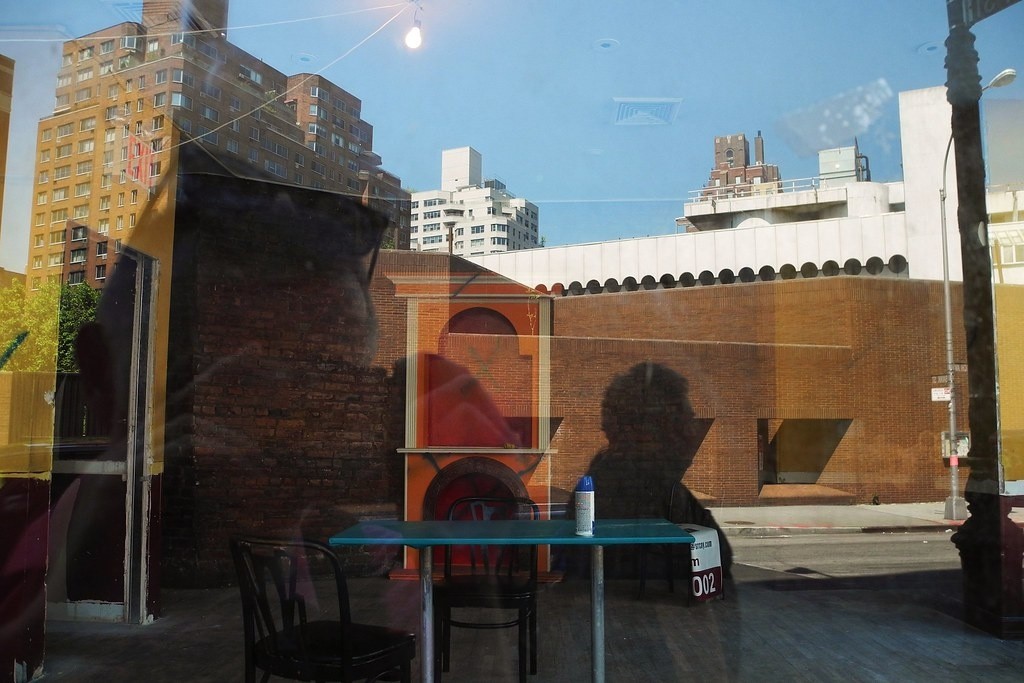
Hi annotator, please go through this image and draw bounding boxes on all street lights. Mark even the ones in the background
[940,69,1018,521]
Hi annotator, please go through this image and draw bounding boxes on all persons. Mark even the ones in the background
[0,211,543,683]
[554,364,739,683]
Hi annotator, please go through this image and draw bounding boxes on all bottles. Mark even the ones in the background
[574,475,596,536]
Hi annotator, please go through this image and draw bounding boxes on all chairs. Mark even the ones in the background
[434,496,542,683]
[227,530,422,683]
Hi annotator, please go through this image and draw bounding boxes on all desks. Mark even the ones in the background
[328,519,696,683]
[633,523,725,609]
